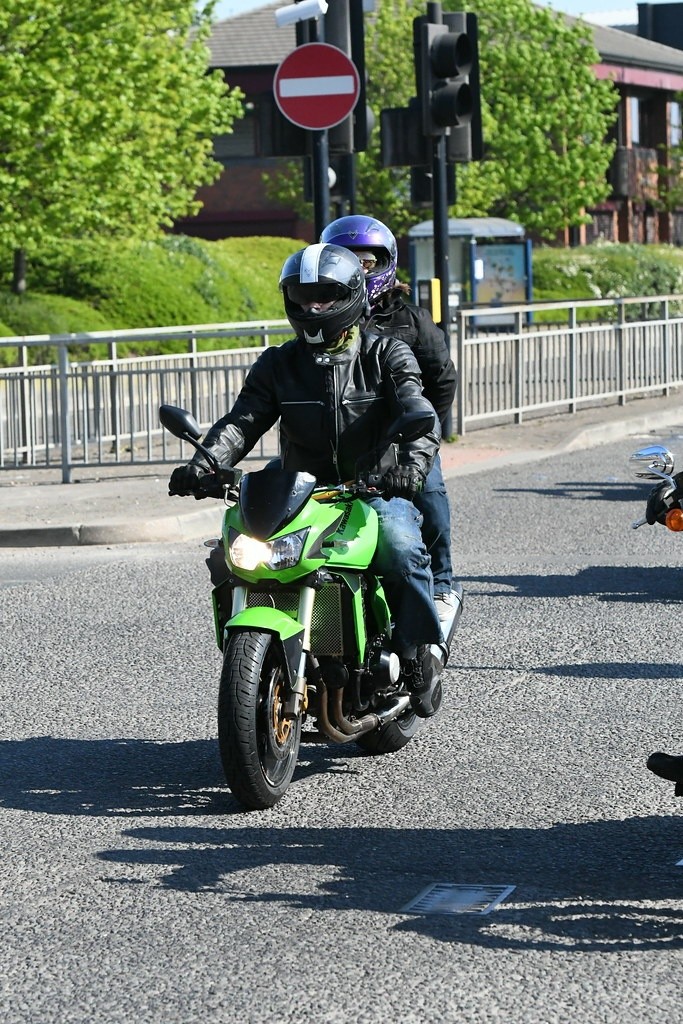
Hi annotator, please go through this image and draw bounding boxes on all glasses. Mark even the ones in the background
[288,283,349,305]
[357,253,381,270]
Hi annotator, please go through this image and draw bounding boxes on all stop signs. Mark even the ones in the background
[271,42,361,132]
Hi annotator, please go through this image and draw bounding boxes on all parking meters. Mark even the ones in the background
[417,276,443,328]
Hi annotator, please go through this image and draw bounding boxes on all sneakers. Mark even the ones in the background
[402,639,443,719]
[431,593,453,621]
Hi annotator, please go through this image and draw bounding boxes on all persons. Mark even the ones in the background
[317,214,458,623]
[170,243,445,718]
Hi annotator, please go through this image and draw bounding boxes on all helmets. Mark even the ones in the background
[319,215,398,302]
[280,244,367,344]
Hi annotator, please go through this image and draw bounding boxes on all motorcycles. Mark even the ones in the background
[157,402,461,813]
[626,444,683,534]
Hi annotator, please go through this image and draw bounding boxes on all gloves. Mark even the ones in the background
[380,463,423,502]
[168,463,210,500]
[646,471,683,531]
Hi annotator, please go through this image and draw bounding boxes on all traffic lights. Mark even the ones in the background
[420,20,476,139]
